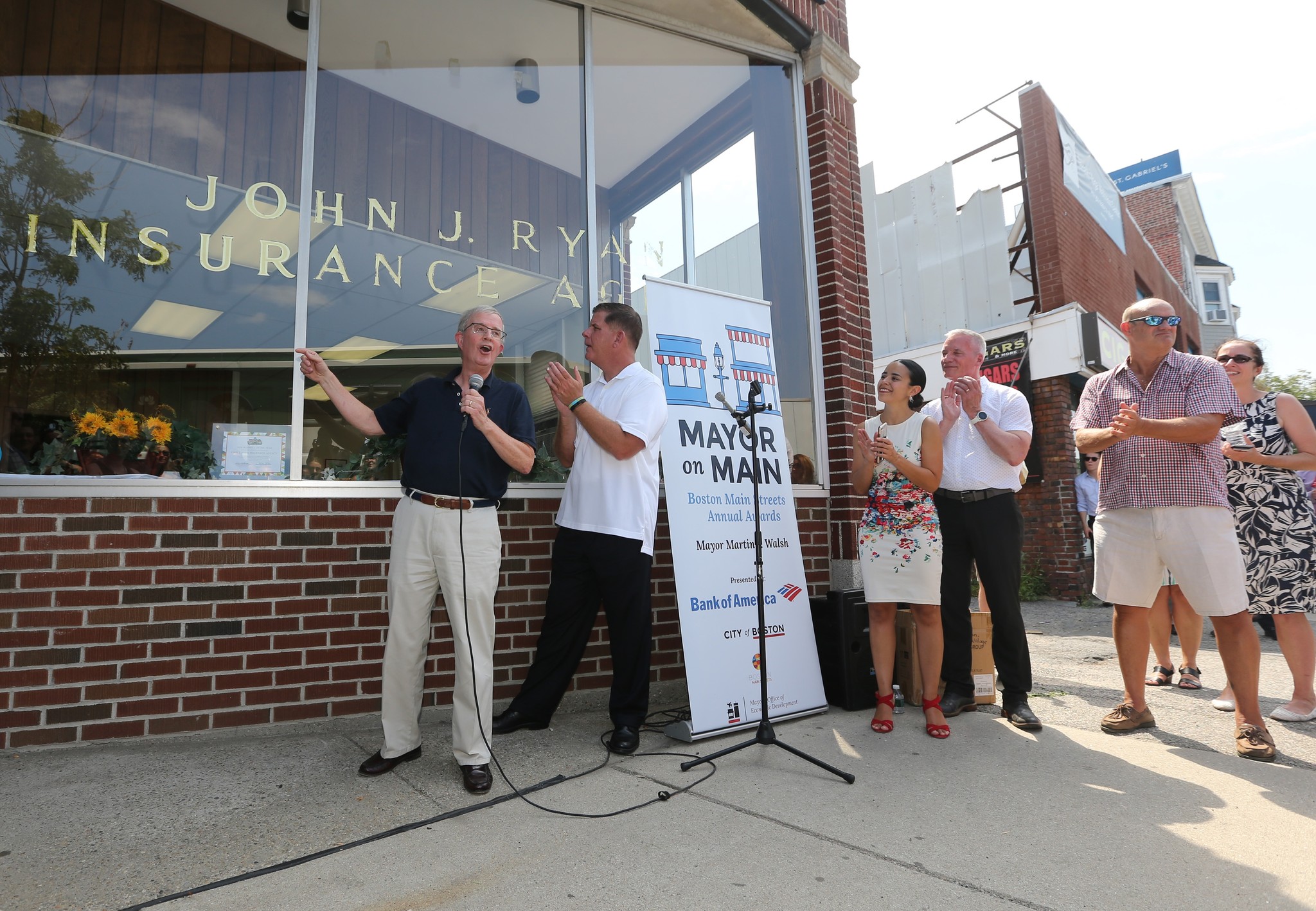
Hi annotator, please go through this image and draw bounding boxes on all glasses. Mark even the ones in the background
[462,323,508,342]
[1084,456,1100,462]
[1212,353,1254,365]
[1125,314,1182,326]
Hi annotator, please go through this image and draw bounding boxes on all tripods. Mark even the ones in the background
[680,379,856,784]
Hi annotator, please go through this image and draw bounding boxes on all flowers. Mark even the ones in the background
[73,401,174,444]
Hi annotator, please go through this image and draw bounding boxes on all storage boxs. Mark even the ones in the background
[895,609,996,706]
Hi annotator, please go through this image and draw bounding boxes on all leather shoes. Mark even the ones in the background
[609,725,640,754]
[459,764,493,794]
[1001,700,1042,728]
[358,743,421,777]
[939,693,978,716]
[492,708,549,734]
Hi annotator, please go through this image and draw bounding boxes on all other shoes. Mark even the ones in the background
[1270,703,1316,721]
[1233,723,1276,762]
[1100,702,1156,733]
[1212,696,1237,711]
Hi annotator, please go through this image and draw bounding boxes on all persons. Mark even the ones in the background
[917,328,1043,730]
[1214,338,1316,721]
[294,306,537,794]
[1296,470,1316,512]
[974,558,991,612]
[785,437,794,479]
[850,359,951,738]
[490,302,668,755]
[792,454,814,485]
[1073,297,1278,762]
[1074,451,1114,607]
[0,404,250,480]
[302,403,398,482]
[1143,567,1202,690]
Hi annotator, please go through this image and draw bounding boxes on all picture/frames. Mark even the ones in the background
[324,459,347,469]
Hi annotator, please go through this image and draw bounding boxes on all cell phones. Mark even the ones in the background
[876,422,888,464]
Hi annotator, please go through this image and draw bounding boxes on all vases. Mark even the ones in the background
[77,447,171,476]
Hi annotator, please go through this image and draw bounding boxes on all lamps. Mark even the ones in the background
[515,58,540,104]
[287,0,309,31]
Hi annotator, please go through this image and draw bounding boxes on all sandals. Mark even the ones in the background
[1179,663,1201,689]
[1144,662,1174,686]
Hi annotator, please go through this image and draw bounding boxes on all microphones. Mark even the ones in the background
[715,392,752,439]
[461,373,484,432]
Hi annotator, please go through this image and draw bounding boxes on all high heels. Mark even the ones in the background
[922,693,950,738]
[871,690,895,733]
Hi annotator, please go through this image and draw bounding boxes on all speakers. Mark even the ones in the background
[811,588,897,711]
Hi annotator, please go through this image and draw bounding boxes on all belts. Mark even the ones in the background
[933,485,1008,503]
[403,486,499,512]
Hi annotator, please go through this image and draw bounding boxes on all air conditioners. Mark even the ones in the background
[1208,309,1228,322]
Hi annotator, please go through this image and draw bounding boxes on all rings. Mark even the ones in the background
[1227,455,1231,457]
[966,389,970,392]
[945,396,949,398]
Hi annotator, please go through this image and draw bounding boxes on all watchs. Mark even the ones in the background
[970,410,988,426]
